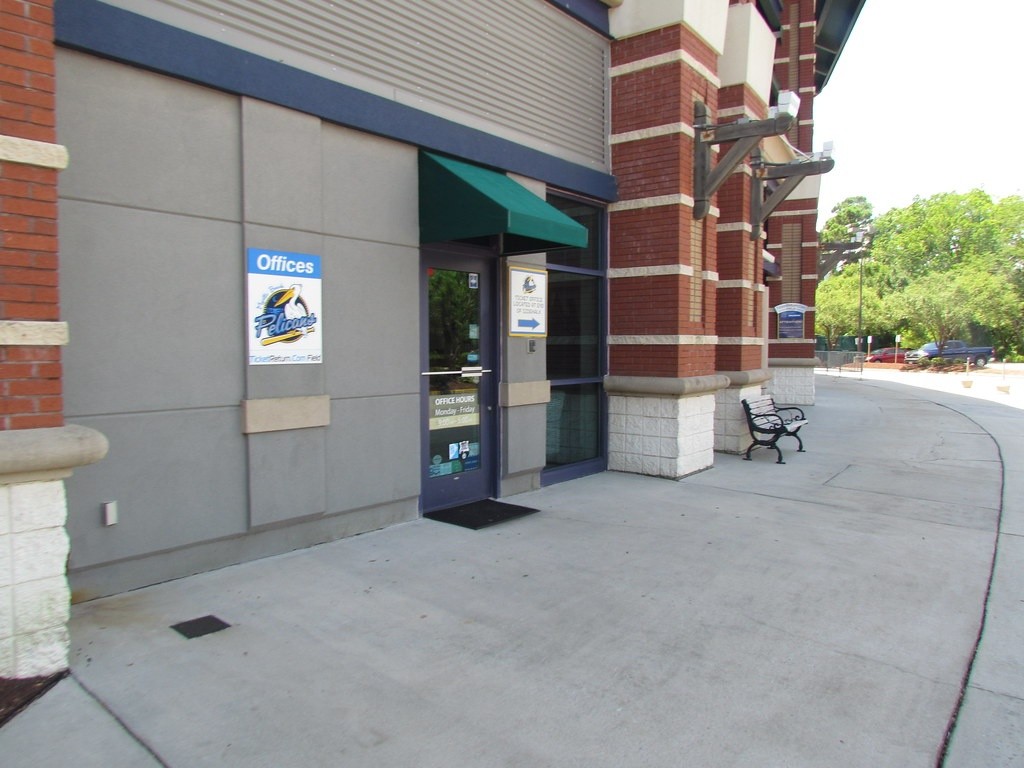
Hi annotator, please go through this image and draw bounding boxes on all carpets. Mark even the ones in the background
[423,498,542,531]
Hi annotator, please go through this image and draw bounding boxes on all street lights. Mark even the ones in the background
[847,222,876,352]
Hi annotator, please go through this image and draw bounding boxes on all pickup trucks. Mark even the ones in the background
[904,340,993,367]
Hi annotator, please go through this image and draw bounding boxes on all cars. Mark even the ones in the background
[865,347,907,363]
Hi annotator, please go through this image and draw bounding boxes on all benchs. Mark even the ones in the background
[741,394,808,465]
